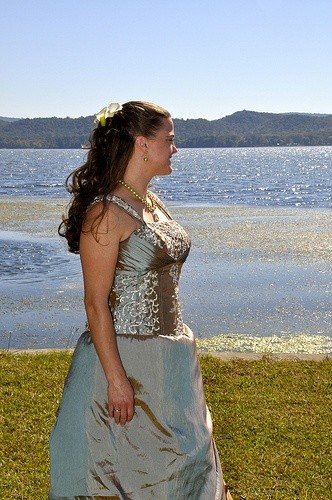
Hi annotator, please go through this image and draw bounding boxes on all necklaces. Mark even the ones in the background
[118,177,159,224]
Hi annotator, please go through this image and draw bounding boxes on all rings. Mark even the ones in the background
[113,408,120,413]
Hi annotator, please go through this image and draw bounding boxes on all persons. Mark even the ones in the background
[46,102,232,500]
